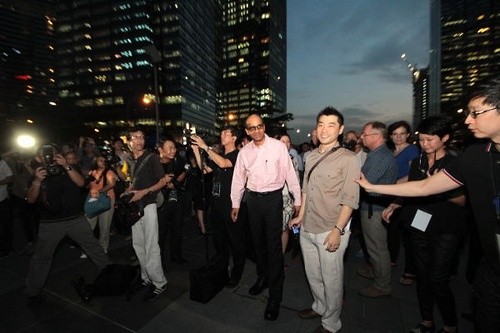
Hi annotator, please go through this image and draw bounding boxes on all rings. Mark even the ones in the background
[334,248,337,249]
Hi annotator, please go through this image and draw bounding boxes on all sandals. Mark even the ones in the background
[398,273,417,288]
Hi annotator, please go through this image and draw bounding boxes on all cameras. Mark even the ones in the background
[40,145,63,176]
[292,224,299,235]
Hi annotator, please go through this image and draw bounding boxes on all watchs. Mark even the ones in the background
[334,226,345,235]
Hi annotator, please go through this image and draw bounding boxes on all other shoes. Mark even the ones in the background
[298,308,322,319]
[358,285,392,298]
[437,327,459,333]
[409,320,435,333]
[139,281,168,298]
[249,275,268,295]
[461,313,474,322]
[26,295,42,305]
[357,268,373,280]
[264,295,280,321]
[313,325,344,333]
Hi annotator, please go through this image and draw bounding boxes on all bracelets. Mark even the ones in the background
[206,146,211,151]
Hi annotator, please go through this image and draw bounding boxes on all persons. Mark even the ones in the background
[0,78,500,333]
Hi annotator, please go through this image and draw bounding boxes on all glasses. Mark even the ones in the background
[464,107,498,119]
[246,124,263,132]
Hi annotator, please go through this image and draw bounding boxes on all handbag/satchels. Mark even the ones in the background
[84,187,111,218]
[113,193,144,234]
[190,260,230,304]
